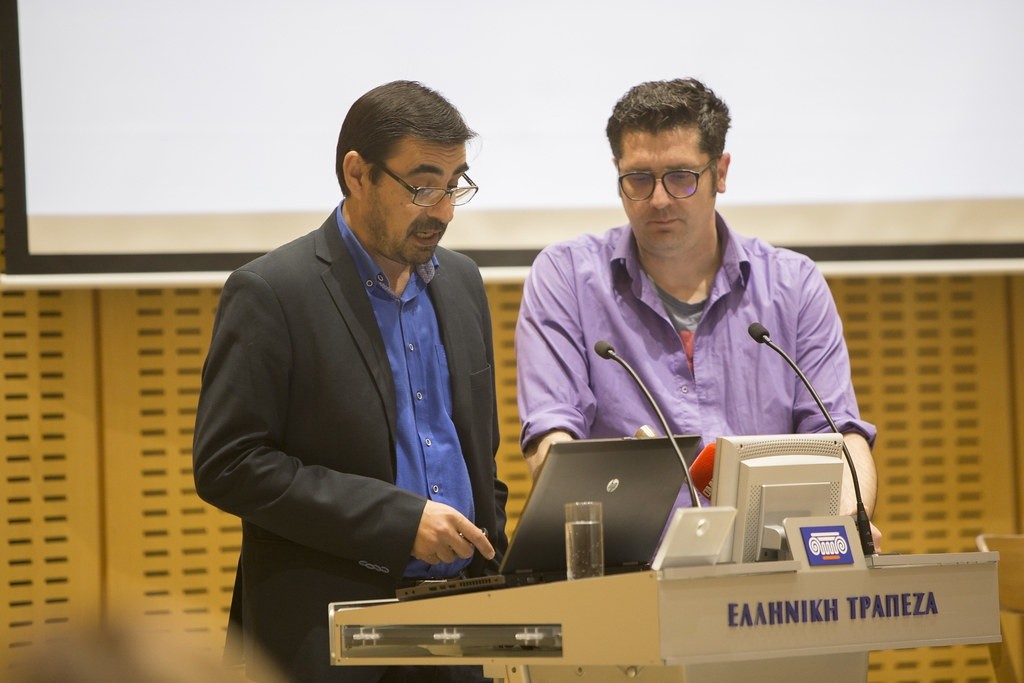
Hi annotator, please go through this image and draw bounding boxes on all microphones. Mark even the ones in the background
[747,322,876,556]
[594,339,704,510]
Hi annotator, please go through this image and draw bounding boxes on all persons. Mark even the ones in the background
[192,80,511,683]
[516,78,883,557]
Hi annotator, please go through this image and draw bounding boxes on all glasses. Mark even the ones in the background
[614,157,716,202]
[376,162,478,207]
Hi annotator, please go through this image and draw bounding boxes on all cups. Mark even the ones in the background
[564,501,605,581]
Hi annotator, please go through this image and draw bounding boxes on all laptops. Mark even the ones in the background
[395,436,702,602]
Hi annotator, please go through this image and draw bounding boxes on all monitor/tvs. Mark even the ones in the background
[715,433,847,569]
